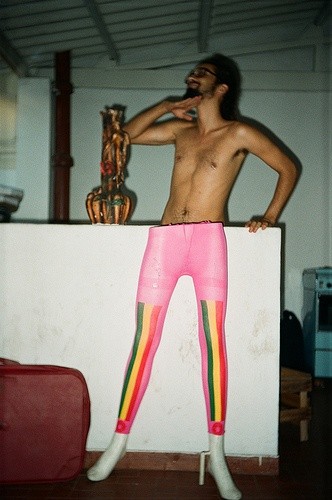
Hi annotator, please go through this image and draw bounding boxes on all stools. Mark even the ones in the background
[280,368,312,440]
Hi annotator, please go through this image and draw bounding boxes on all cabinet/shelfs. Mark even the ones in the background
[302,267,332,378]
